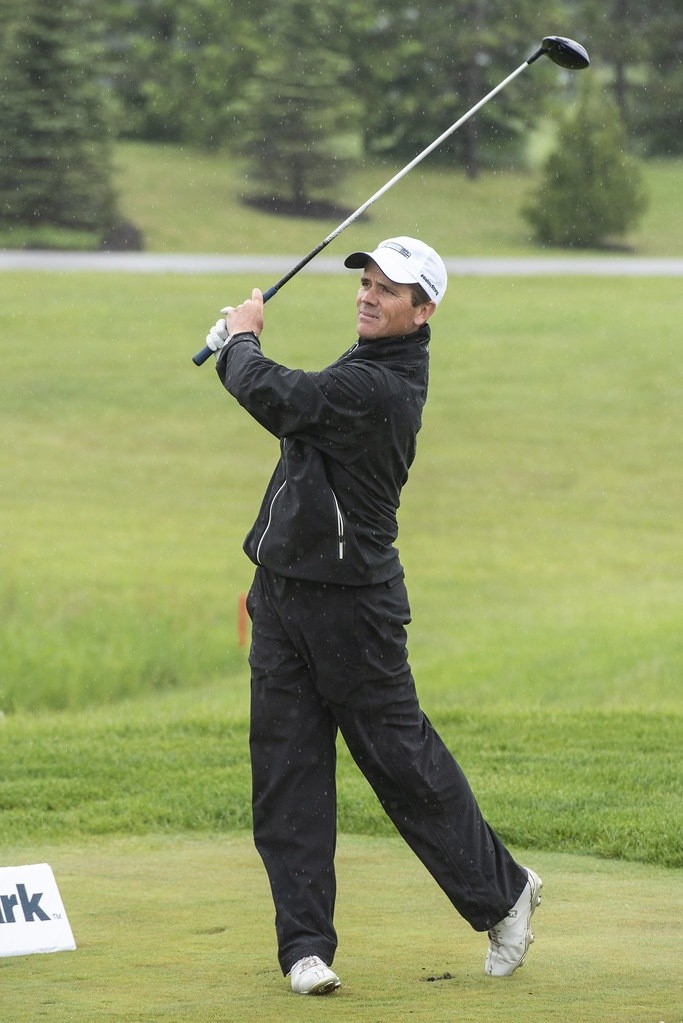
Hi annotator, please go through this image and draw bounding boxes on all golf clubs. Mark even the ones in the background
[191,32,593,369]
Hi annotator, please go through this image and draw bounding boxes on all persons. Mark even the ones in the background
[213,237,544,994]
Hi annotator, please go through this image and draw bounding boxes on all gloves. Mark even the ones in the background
[207,306,234,363]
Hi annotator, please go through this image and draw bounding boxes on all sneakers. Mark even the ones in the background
[289,953,341,996]
[484,866,543,980]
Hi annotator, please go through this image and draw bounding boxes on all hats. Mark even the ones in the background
[345,236,446,307]
